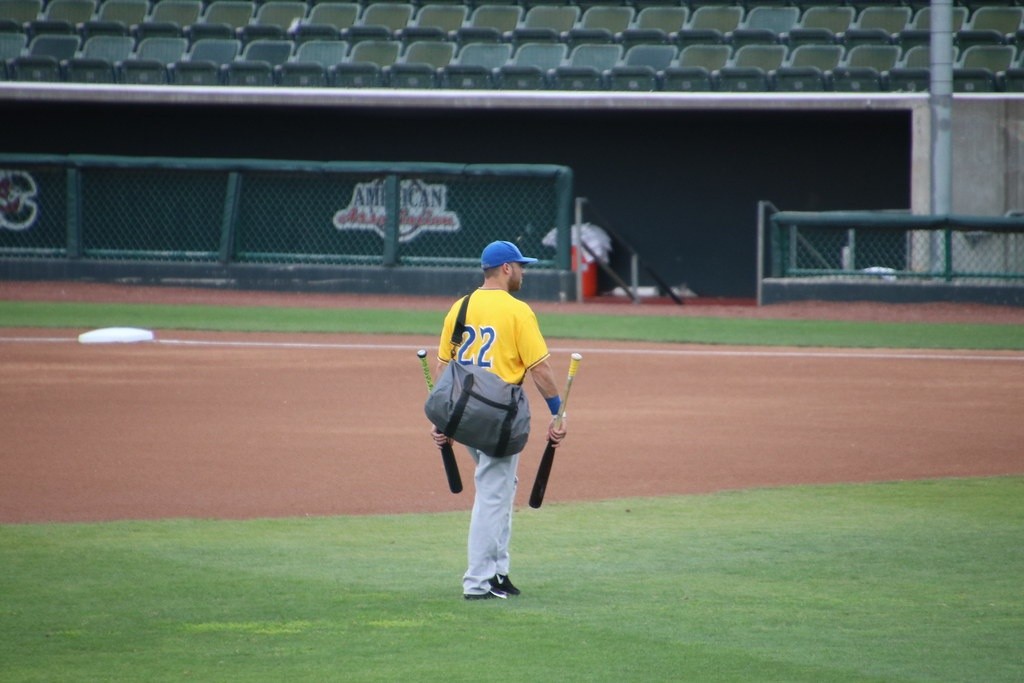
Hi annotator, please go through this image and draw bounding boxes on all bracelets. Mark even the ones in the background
[546,396,561,415]
[552,411,566,419]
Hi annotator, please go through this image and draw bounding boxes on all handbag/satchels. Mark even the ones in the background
[424,361,532,457]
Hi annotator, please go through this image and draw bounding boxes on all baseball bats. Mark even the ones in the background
[416,348,464,495]
[528,352,583,509]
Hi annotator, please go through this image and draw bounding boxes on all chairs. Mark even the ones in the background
[0,0,1024,104]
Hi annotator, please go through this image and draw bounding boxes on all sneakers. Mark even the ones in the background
[488,572,521,596]
[464,586,510,601]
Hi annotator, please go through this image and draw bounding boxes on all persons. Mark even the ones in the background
[431,240,567,601]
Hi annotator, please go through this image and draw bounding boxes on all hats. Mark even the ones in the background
[481,240,538,270]
